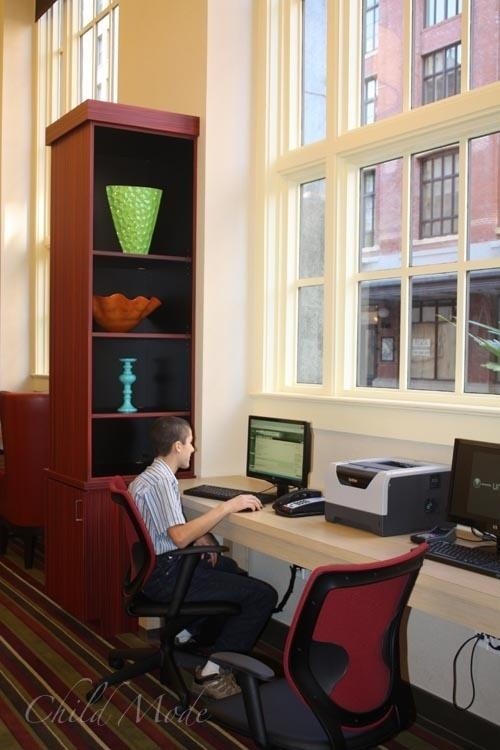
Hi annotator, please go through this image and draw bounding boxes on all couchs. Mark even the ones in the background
[0,391,50,571]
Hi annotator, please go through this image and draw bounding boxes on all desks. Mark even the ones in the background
[171,476,500,634]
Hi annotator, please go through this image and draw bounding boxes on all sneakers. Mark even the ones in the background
[190,663,243,701]
[173,635,201,655]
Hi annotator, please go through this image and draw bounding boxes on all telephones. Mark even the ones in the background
[271,489,326,518]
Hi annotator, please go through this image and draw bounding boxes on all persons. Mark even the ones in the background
[127,417,277,700]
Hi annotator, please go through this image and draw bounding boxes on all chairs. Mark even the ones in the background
[203,542,432,749]
[85,477,240,718]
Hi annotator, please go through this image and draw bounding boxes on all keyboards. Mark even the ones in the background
[413,538,499,579]
[183,484,276,505]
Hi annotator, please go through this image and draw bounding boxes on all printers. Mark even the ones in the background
[323,455,451,538]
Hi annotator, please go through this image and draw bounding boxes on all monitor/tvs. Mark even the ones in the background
[244,415,314,496]
[443,436,499,554]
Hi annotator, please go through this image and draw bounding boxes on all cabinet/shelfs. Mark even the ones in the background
[41,99,201,650]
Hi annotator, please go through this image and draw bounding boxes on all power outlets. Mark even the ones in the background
[289,563,305,580]
[473,630,500,654]
